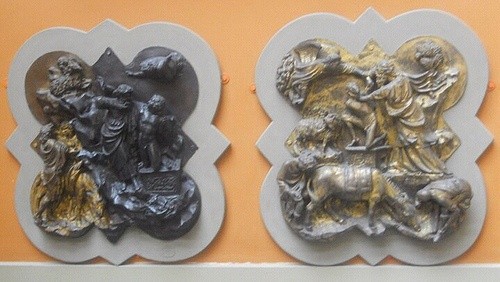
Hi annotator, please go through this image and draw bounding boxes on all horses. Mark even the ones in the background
[303,162,417,232]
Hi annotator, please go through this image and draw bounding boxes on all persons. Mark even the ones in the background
[281,37,473,243]
[33,52,202,240]
